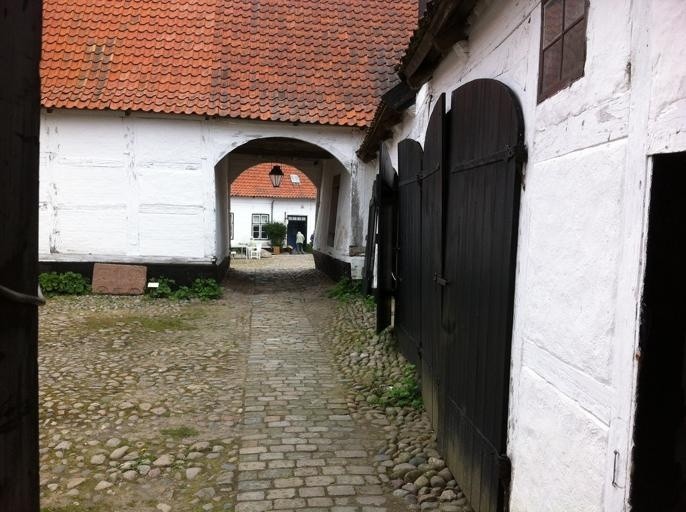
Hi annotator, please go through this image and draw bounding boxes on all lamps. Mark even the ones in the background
[267,155,285,188]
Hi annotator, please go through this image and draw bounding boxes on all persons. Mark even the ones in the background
[295,230,305,254]
